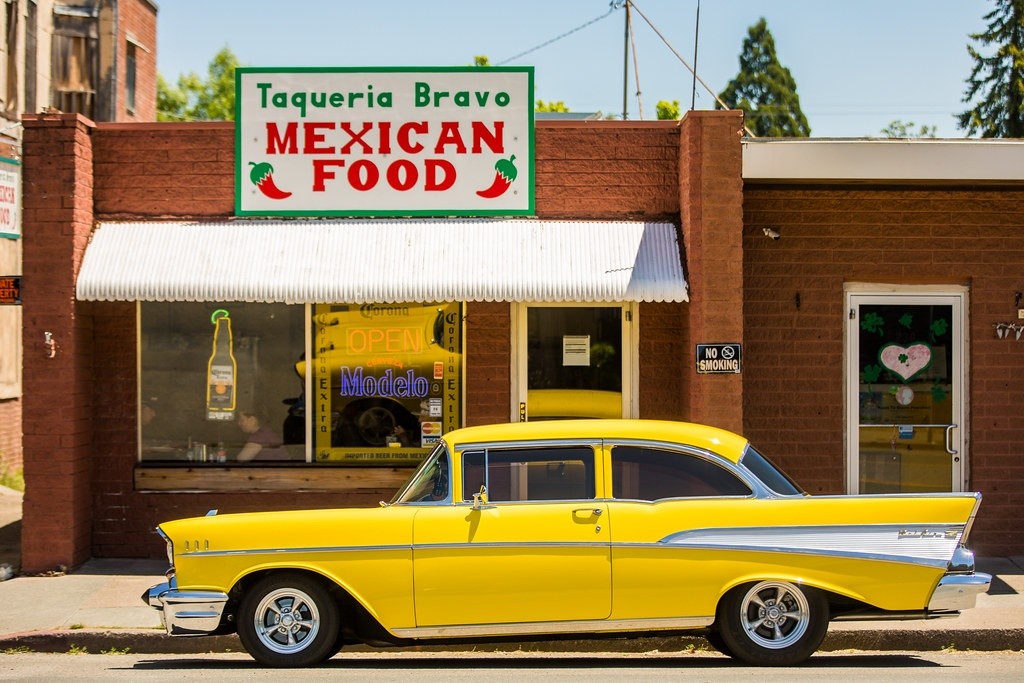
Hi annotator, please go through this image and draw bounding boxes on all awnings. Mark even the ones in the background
[77,218,689,305]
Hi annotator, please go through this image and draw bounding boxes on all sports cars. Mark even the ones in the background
[142,417,997,669]
[282,308,624,450]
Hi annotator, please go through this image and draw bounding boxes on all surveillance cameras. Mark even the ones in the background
[763,228,780,241]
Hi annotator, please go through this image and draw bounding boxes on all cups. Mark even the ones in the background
[386,437,396,447]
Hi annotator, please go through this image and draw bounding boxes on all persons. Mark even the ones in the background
[140,394,287,462]
[393,401,437,447]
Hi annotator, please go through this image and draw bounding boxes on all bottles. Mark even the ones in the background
[207,317,237,420]
[216,442,225,460]
[186,434,193,459]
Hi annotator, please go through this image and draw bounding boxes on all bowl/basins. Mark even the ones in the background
[388,443,401,448]
